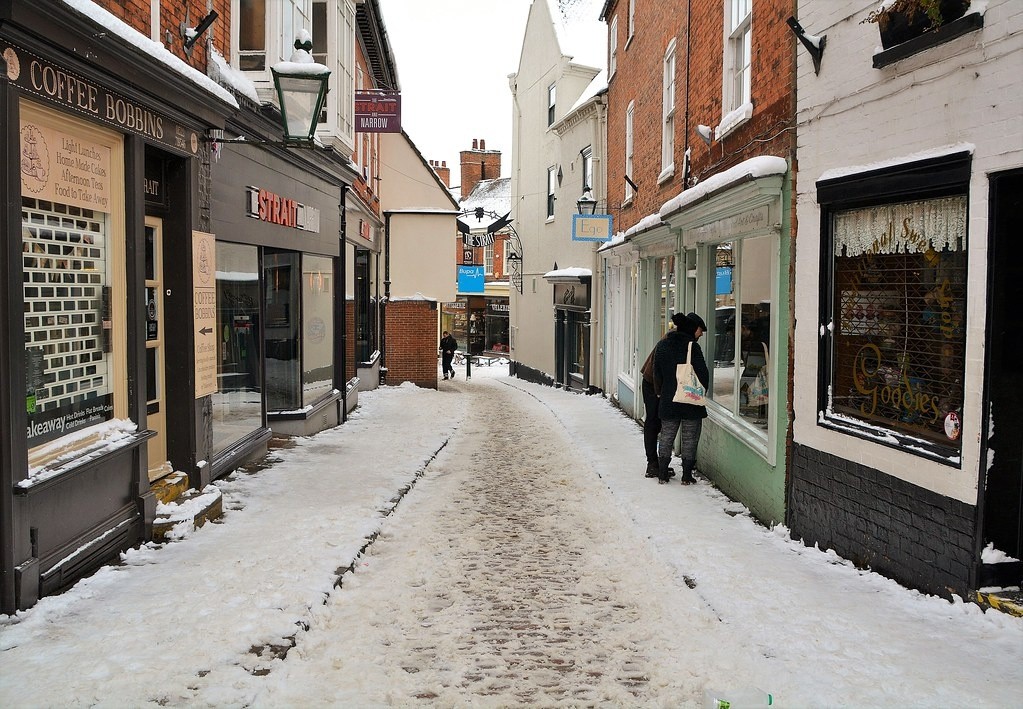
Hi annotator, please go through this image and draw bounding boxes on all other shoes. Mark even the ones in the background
[441,376,448,380]
[450,370,455,379]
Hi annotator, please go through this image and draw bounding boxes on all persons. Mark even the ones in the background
[640,312,708,478]
[730,314,772,426]
[652,313,710,485]
[439,331,458,379]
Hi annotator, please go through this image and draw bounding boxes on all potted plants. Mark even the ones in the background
[859,0,971,50]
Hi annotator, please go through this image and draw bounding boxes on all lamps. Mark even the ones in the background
[198,29,331,151]
[577,186,621,216]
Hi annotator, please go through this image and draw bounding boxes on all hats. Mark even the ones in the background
[671,313,687,333]
[686,312,708,333]
[725,314,750,328]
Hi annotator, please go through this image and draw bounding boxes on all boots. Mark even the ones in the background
[643,433,676,478]
[658,457,677,484]
[681,459,697,485]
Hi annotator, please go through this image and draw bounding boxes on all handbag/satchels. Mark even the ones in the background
[747,342,769,407]
[673,342,707,406]
